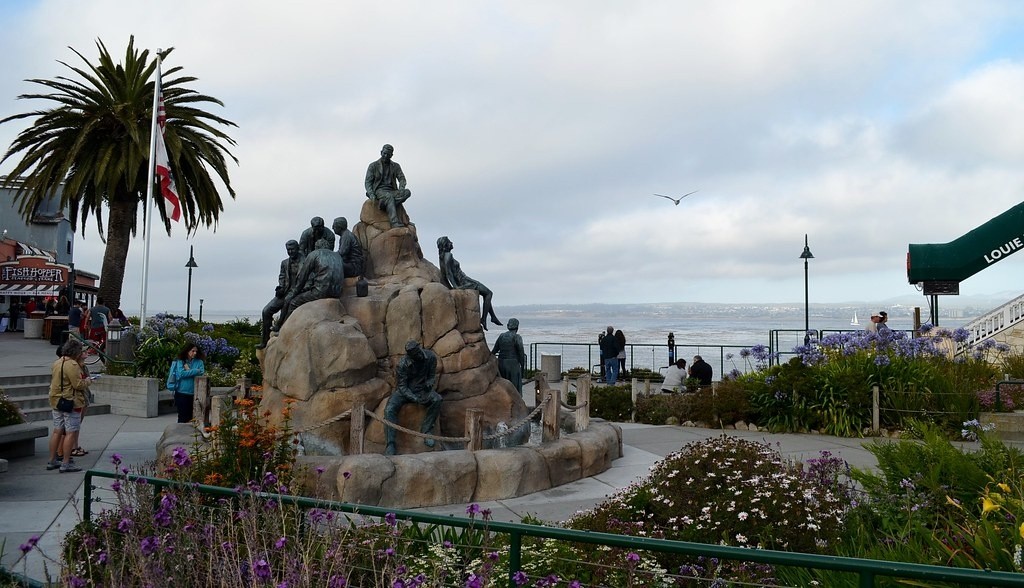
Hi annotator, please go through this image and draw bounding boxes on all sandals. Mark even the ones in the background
[77,447,88,454]
[70,449,85,456]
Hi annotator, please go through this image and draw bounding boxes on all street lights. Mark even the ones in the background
[798,234,815,347]
[200,299,204,323]
[185,244,199,322]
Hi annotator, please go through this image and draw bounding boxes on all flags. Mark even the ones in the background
[156,66,181,223]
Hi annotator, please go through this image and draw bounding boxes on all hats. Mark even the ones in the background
[81,346,90,351]
[871,311,883,317]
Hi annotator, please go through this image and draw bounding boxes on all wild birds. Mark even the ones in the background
[653,191,698,205]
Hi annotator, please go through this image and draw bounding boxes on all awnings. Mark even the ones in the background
[0,284,64,296]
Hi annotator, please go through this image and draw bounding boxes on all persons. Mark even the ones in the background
[661,358,687,393]
[299,217,335,258]
[865,312,883,335]
[491,318,524,399]
[254,240,317,350]
[438,236,503,331]
[174,342,205,423]
[877,311,888,333]
[688,355,713,393]
[282,237,345,319]
[365,144,411,228]
[617,329,626,382]
[46,339,98,473]
[384,340,443,455]
[6,294,126,342]
[332,217,365,278]
[600,326,620,386]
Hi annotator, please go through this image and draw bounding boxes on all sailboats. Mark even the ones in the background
[850,312,860,326]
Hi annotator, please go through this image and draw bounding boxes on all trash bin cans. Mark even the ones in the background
[50,319,69,345]
[24,319,43,338]
[541,354,561,383]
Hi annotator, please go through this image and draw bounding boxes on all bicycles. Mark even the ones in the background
[82,332,103,365]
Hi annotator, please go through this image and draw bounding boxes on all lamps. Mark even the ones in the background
[107,320,122,341]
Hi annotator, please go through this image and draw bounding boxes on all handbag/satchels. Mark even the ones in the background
[57,397,75,413]
[167,362,178,390]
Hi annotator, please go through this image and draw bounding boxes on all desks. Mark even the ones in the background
[44,315,92,320]
[0,318,25,332]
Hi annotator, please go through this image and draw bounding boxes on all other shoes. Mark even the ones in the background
[60,464,81,471]
[47,462,60,469]
[9,329,12,332]
[56,455,74,464]
[14,330,16,332]
